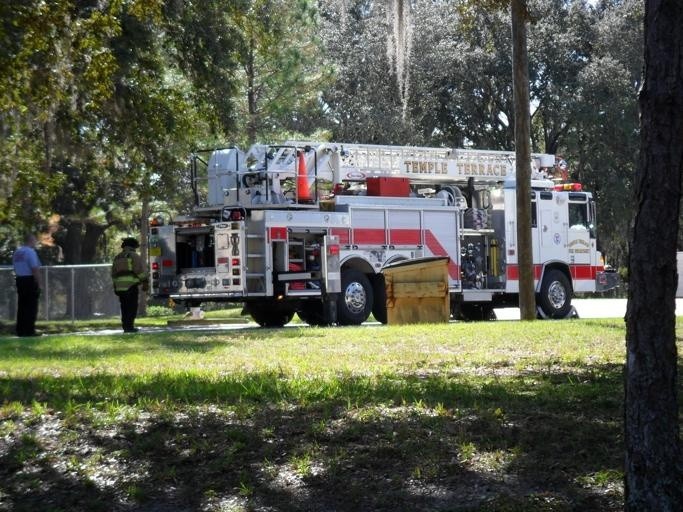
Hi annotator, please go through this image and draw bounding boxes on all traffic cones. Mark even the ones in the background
[292,152,311,201]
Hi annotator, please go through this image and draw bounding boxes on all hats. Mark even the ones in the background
[121,237,140,248]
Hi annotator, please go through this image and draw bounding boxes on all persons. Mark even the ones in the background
[110,238,150,332]
[11,233,44,337]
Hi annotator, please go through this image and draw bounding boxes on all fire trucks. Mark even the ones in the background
[147,139,621,332]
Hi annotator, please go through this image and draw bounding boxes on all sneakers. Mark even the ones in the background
[124,328,139,332]
